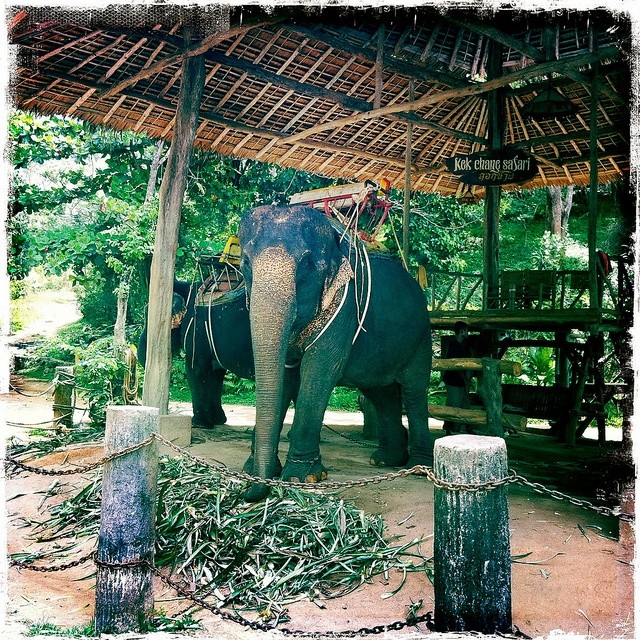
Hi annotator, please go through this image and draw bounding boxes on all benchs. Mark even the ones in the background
[198,236,241,293]
[283,176,392,243]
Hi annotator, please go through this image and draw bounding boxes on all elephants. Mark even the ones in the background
[236,203,435,502]
[137,253,324,442]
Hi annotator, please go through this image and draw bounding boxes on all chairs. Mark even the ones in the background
[485,269,524,310]
[512,268,557,310]
